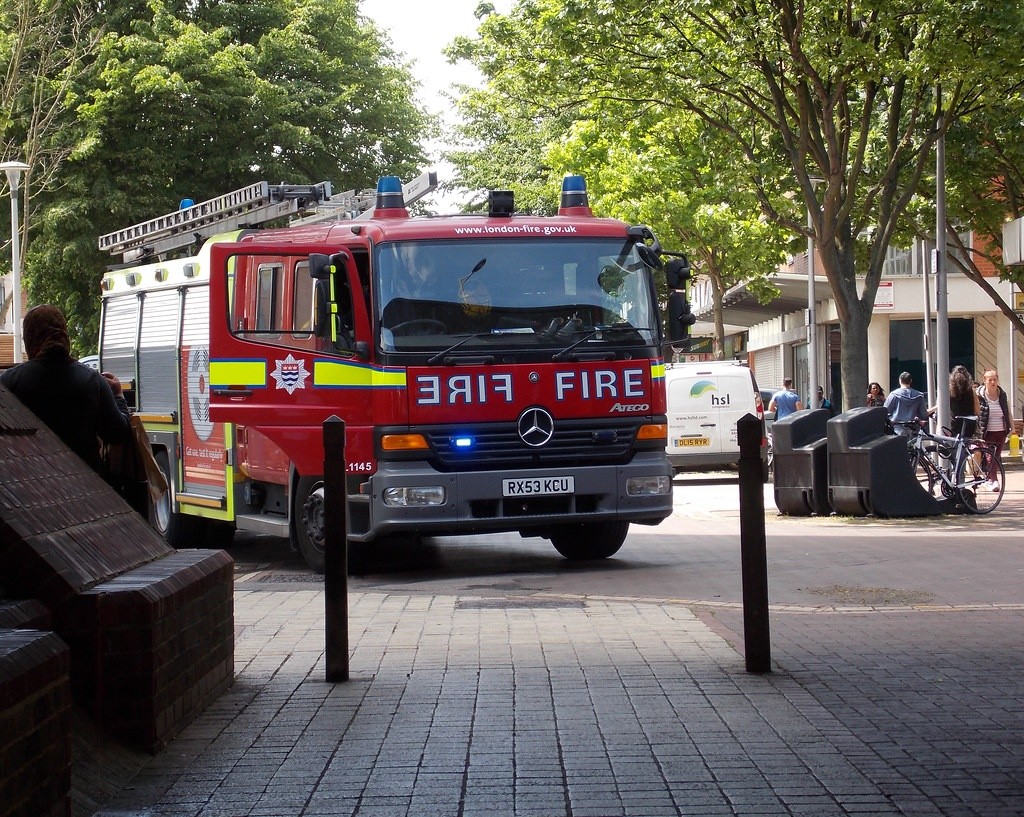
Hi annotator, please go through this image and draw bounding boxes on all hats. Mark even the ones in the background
[818,386,823,394]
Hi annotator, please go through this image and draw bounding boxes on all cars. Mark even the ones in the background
[79,353,98,372]
[762,386,804,434]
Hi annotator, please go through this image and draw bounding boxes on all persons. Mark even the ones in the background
[949,366,980,485]
[0,305,132,479]
[767,377,804,420]
[976,370,1015,492]
[806,386,832,409]
[884,371,937,435]
[867,383,886,407]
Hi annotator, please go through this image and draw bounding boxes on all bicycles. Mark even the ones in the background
[892,413,1006,515]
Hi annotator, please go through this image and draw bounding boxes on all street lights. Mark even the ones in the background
[0,159,38,362]
[795,171,823,409]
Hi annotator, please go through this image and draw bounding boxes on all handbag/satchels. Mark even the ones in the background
[123,413,168,507]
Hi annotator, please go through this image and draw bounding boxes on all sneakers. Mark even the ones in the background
[993,481,999,492]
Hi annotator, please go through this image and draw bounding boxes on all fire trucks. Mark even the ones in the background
[88,172,698,571]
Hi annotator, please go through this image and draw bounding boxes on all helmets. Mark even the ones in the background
[458,273,493,316]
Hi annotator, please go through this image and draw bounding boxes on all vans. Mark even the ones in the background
[664,361,768,482]
